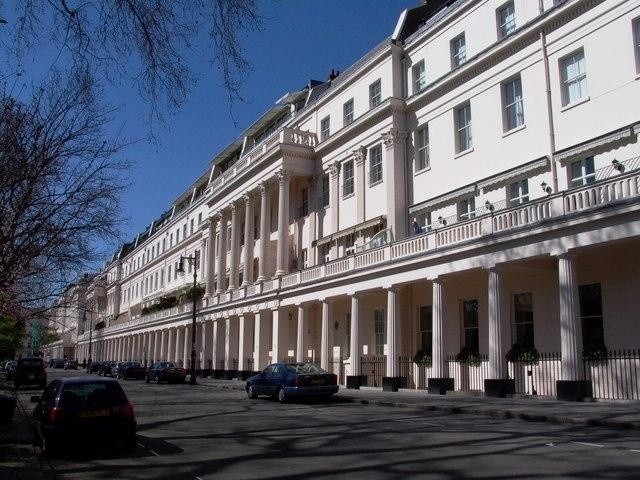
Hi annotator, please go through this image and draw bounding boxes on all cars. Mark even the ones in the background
[144,360,187,385]
[87,360,146,381]
[31,376,137,460]
[244,362,339,404]
[1,360,17,379]
[49,358,79,372]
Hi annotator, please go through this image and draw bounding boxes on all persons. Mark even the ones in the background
[410,217,420,236]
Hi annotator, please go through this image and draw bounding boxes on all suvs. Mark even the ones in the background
[13,357,47,389]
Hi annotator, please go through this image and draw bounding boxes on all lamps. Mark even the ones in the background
[438,216,446,224]
[611,158,621,171]
[541,181,549,192]
[485,200,492,211]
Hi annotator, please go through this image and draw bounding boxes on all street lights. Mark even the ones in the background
[82,305,96,374]
[176,249,198,386]
